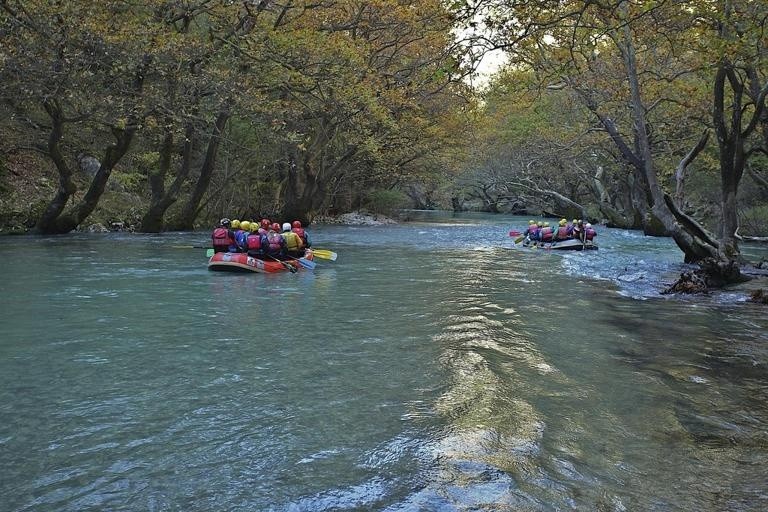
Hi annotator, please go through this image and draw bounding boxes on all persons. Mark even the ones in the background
[523,218,597,243]
[212,215,312,261]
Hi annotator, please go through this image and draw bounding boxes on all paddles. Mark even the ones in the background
[266,254,297,273]
[286,254,316,270]
[510,231,523,236]
[515,235,526,244]
[531,240,552,251]
[207,249,215,257]
[300,249,338,261]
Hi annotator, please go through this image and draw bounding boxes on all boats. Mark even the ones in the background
[208,247,313,273]
[522,233,600,253]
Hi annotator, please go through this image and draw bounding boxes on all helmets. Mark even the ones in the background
[528,218,592,229]
[220,218,302,231]
